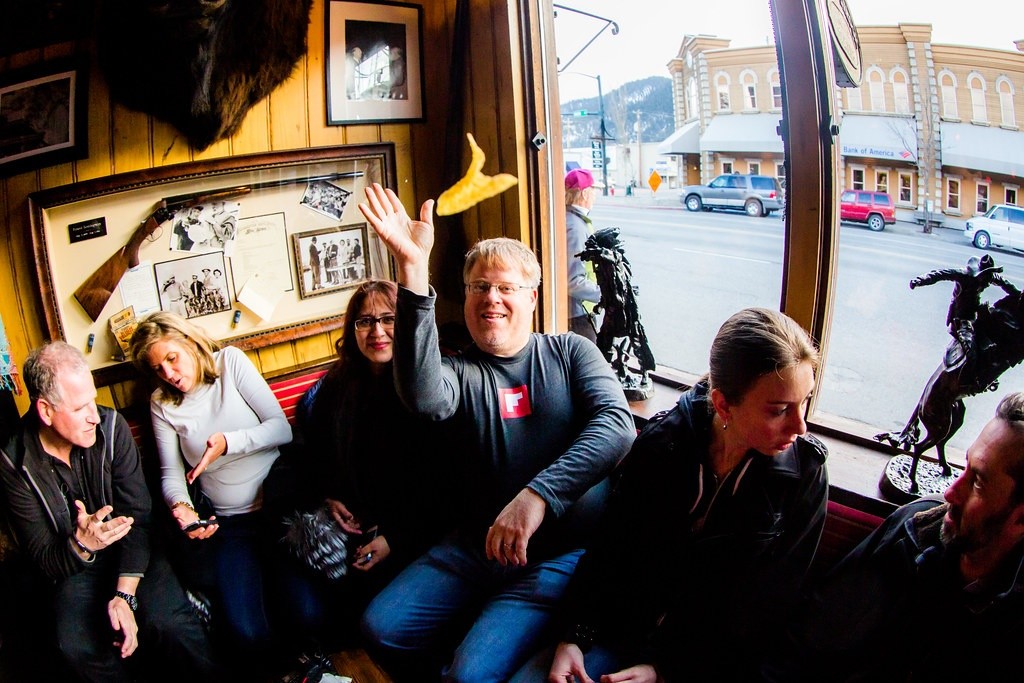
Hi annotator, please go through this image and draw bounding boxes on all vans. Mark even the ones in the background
[964,204,1024,250]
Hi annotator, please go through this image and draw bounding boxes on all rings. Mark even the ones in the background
[205,468,207,470]
[504,544,512,547]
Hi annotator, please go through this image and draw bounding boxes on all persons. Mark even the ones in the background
[185,202,236,253]
[0,341,210,683]
[911,254,1024,353]
[309,237,362,291]
[383,47,404,87]
[577,226,656,383]
[346,47,363,100]
[513,307,1024,683]
[565,169,602,344]
[173,206,204,251]
[161,268,225,317]
[130,310,326,639]
[359,182,637,683]
[261,280,428,572]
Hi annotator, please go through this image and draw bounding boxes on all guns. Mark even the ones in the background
[73,171,364,323]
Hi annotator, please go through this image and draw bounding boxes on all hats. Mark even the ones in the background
[565,169,606,189]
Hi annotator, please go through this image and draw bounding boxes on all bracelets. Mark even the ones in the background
[174,502,194,512]
[72,531,98,554]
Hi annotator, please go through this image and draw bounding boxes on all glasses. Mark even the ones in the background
[353,315,396,331]
[465,280,534,297]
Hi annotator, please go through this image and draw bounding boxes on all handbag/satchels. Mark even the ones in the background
[278,500,381,580]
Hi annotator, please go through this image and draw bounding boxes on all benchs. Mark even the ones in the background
[121,370,884,588]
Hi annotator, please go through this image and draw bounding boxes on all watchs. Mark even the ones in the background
[115,591,138,611]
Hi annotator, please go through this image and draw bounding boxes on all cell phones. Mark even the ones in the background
[182,519,219,532]
[358,525,379,565]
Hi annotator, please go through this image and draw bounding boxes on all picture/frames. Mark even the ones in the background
[153,250,232,320]
[292,222,373,302]
[27,142,401,391]
[0,53,92,181]
[324,0,426,127]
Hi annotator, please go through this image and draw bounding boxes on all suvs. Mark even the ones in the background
[841,190,896,232]
[679,174,783,217]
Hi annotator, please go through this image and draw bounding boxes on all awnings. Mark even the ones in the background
[839,115,918,161]
[701,115,784,153]
[656,121,700,154]
[940,120,1024,178]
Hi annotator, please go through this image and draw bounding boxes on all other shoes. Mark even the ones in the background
[297,647,337,681]
